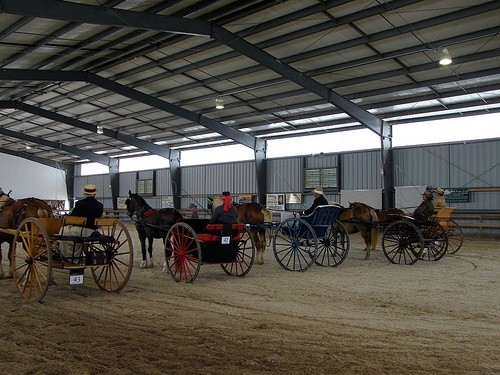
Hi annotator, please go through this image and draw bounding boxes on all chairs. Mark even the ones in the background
[91,218,117,264]
[432,207,455,232]
[229,224,245,240]
[198,224,224,242]
[54,216,87,266]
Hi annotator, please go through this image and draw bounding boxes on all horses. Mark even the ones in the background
[327,202,379,262]
[347,200,407,252]
[205,191,272,265]
[123,188,185,274]
[0,186,55,289]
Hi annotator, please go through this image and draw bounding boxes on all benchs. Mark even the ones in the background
[301,201,344,235]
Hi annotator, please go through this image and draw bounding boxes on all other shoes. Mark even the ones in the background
[52,252,63,262]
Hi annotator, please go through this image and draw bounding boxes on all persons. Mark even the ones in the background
[52,184,103,265]
[432,188,446,208]
[413,191,434,223]
[209,192,238,224]
[299,186,330,217]
[189,203,199,219]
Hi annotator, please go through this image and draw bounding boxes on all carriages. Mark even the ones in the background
[0,187,135,304]
[205,195,350,273]
[328,200,464,265]
[124,189,256,284]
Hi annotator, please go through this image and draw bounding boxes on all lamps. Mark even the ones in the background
[215,97,224,109]
[26,142,32,149]
[439,48,452,65]
[97,125,103,133]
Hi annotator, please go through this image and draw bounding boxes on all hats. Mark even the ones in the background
[221,191,232,211]
[311,186,324,195]
[187,203,198,209]
[421,191,434,199]
[434,187,444,195]
[80,184,98,194]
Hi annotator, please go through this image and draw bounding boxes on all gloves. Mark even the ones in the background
[297,210,304,216]
